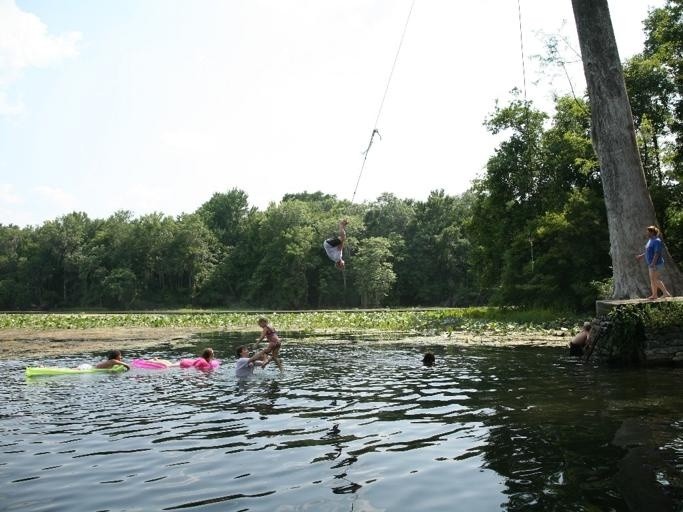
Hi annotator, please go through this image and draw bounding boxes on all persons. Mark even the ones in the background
[95,348,130,371]
[568,323,591,356]
[423,353,435,362]
[235,342,277,377]
[324,219,348,271]
[635,226,671,299]
[255,316,284,373]
[202,347,215,363]
